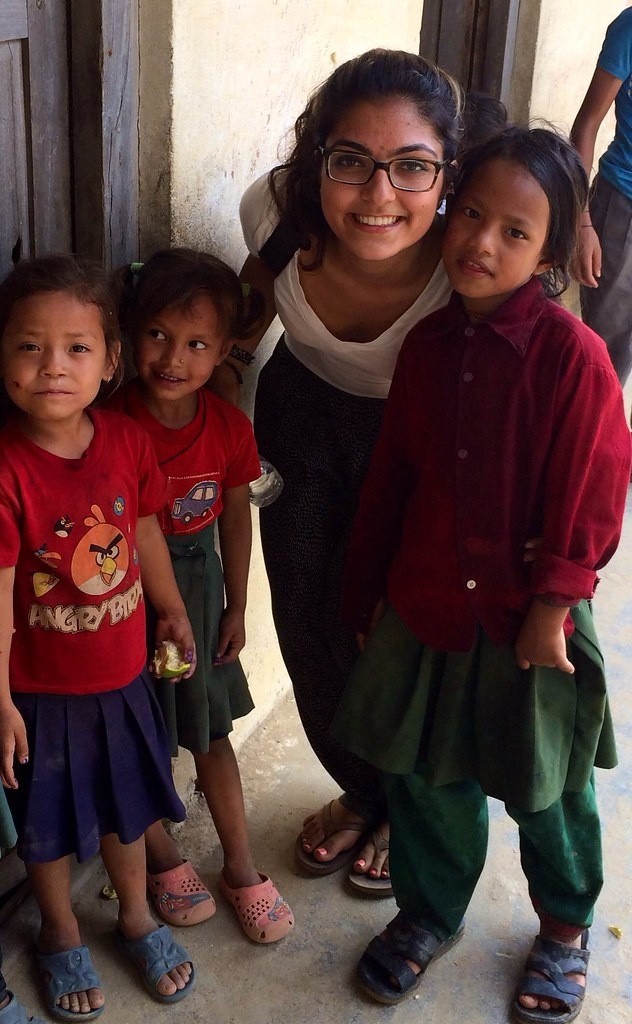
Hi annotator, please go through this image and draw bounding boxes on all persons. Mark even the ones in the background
[330,126,632,1024]
[206,46,508,894]
[110,247,295,946]
[569,6,632,391]
[0,256,197,1019]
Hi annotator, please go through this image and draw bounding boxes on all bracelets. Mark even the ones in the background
[224,361,243,384]
[583,225,593,227]
[229,344,255,366]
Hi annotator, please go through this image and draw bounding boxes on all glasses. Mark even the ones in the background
[318,145,451,192]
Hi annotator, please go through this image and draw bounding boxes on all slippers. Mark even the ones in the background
[0,989,46,1024]
[145,858,215,926]
[35,938,104,1022]
[356,909,466,1003]
[295,792,395,896]
[514,929,590,1024]
[218,866,295,944]
[118,923,196,1005]
